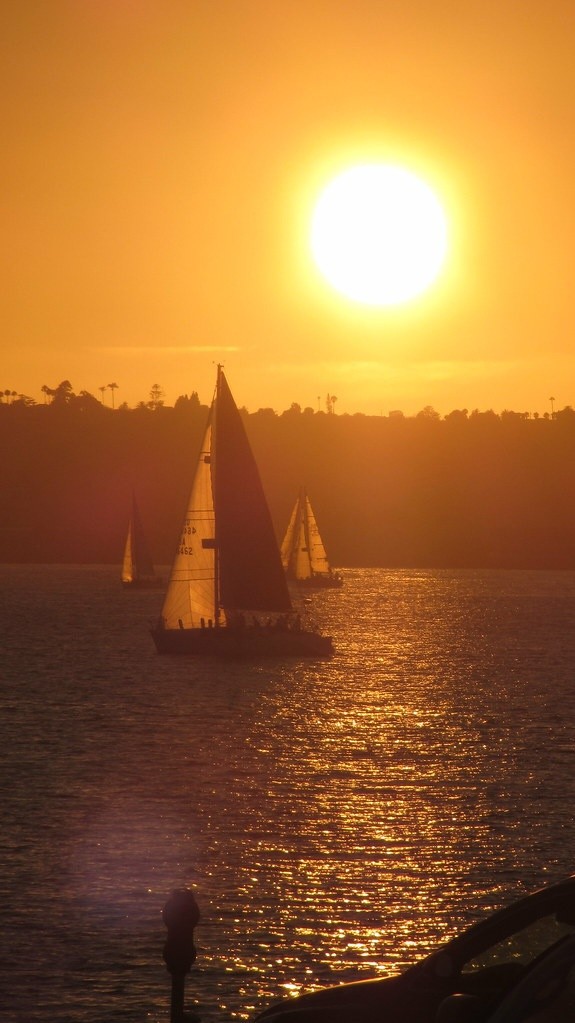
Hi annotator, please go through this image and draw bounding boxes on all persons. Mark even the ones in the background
[315,571,339,580]
[251,615,301,632]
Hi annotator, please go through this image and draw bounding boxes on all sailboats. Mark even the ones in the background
[119,490,166,592]
[147,362,336,657]
[279,483,343,588]
